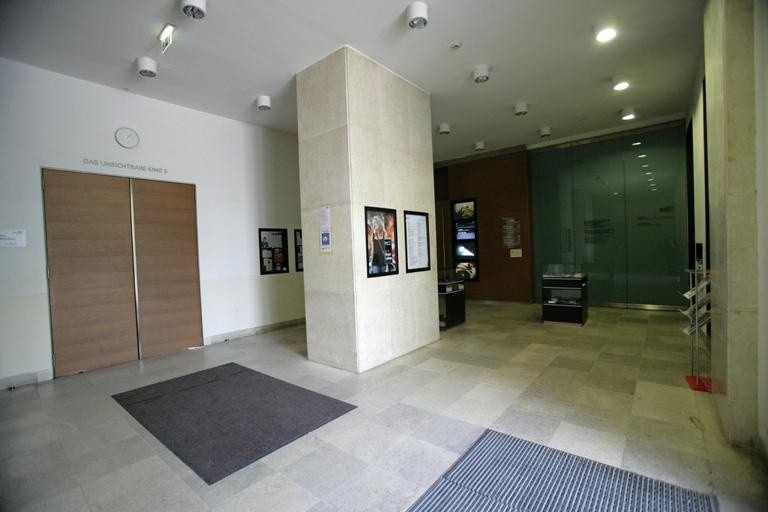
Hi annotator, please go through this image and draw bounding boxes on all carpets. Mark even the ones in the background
[111,362,359,485]
[404,429,722,512]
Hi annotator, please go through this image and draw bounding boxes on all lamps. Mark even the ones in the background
[440,122,450,136]
[181,0,208,20]
[475,142,486,152]
[513,103,528,116]
[539,127,551,137]
[594,19,616,41]
[611,74,628,91]
[138,57,157,80]
[257,96,270,111]
[406,2,428,30]
[473,64,489,83]
[631,135,659,192]
[619,107,636,121]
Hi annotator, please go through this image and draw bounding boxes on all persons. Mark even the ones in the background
[274,248,283,263]
[261,236,268,246]
[370,216,387,274]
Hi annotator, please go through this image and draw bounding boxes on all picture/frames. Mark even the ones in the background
[294,229,303,272]
[365,206,399,278]
[258,228,289,275]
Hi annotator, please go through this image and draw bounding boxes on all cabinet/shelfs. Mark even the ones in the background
[683,269,712,391]
[438,280,465,330]
[541,274,588,326]
